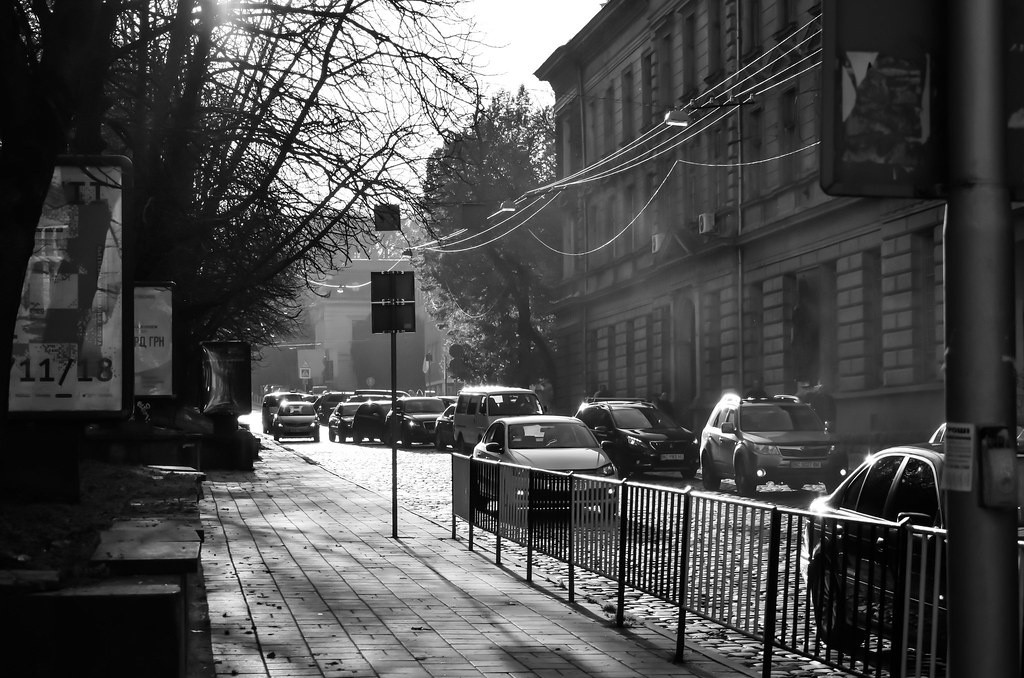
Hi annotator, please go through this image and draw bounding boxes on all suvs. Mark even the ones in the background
[699,388,849,499]
[562,396,699,481]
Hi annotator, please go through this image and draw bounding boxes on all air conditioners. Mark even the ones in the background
[698,212,716,234]
[651,234,664,252]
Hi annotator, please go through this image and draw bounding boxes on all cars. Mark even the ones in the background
[433,402,485,452]
[299,389,459,424]
[269,400,320,444]
[383,397,446,448]
[927,423,1024,461]
[328,401,368,444]
[471,413,622,524]
[804,442,1024,678]
[353,400,395,445]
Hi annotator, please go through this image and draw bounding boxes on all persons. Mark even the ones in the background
[593,384,612,400]
[654,391,681,423]
[743,379,767,398]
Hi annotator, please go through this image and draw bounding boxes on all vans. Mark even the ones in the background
[260,393,304,435]
[454,386,556,454]
